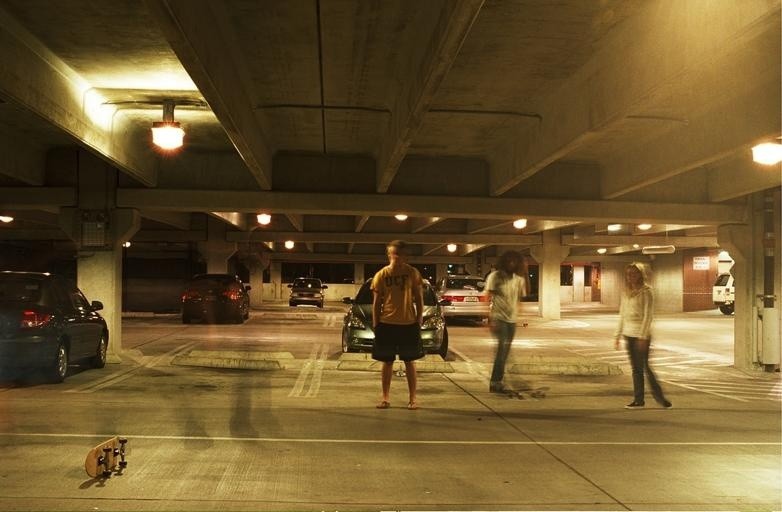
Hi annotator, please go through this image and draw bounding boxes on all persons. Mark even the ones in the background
[613,261,676,410]
[484,249,527,394]
[369,241,425,411]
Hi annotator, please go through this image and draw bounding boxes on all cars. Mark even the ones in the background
[0,271,109,384]
[287,277,328,308]
[341,274,493,359]
[181,273,251,324]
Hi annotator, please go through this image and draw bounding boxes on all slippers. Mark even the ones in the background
[407,402,416,410]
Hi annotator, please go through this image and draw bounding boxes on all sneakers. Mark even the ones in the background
[376,401,391,408]
[662,400,673,408]
[625,401,645,409]
[489,383,512,394]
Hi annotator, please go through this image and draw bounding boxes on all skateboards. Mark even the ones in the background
[498,387,551,399]
[85,436,127,478]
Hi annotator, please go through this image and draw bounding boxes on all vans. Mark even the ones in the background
[712,272,735,315]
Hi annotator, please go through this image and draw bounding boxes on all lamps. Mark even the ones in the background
[749,135,781,168]
[148,101,184,151]
[257,213,272,225]
